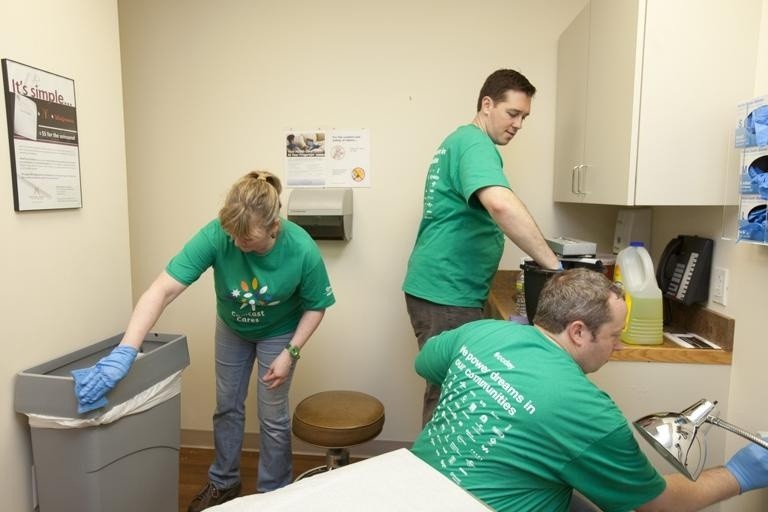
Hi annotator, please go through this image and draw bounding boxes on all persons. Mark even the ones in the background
[400,69,564,431]
[303,139,320,152]
[77,169,337,512]
[410,266,768,511]
[286,134,303,152]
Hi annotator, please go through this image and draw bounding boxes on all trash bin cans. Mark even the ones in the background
[15,331,189,512]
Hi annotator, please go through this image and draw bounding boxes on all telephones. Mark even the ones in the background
[656,234,713,308]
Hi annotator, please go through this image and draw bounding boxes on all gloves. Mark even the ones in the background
[79,346,138,406]
[724,437,767,496]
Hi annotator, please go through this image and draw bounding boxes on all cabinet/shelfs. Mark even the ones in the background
[552,0,763,206]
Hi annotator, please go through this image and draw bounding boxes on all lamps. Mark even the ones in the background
[633,398,768,482]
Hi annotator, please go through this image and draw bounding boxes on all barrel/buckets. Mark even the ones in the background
[613,240,665,345]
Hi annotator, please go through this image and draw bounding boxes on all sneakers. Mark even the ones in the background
[188,481,241,512]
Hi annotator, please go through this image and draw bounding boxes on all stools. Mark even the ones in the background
[292,390,386,482]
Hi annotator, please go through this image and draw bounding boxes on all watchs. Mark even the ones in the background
[284,343,301,360]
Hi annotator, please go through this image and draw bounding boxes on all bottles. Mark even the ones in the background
[514,256,533,318]
